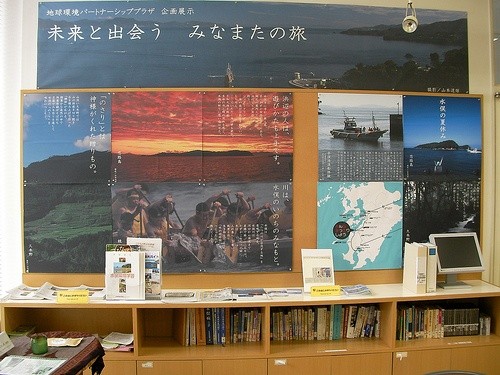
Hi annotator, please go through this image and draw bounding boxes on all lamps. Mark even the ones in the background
[401,1,417,33]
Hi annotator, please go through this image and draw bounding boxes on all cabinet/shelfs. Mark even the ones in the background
[0,280,500,374]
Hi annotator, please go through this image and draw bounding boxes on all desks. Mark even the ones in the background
[0,331,104,375]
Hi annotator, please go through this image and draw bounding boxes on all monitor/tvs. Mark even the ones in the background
[429,232,485,289]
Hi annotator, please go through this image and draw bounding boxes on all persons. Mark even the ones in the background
[112,186,291,270]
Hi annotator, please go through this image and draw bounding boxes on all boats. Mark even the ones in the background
[330,109,389,142]
[165,222,292,267]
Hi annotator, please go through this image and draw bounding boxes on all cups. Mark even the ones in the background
[32,335,48,354]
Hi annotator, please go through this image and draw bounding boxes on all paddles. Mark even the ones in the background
[172,205,185,226]
[197,206,217,266]
[223,194,240,264]
[136,188,151,204]
[162,201,177,262]
[139,205,142,238]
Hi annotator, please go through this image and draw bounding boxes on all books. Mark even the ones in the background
[0,239,491,375]
